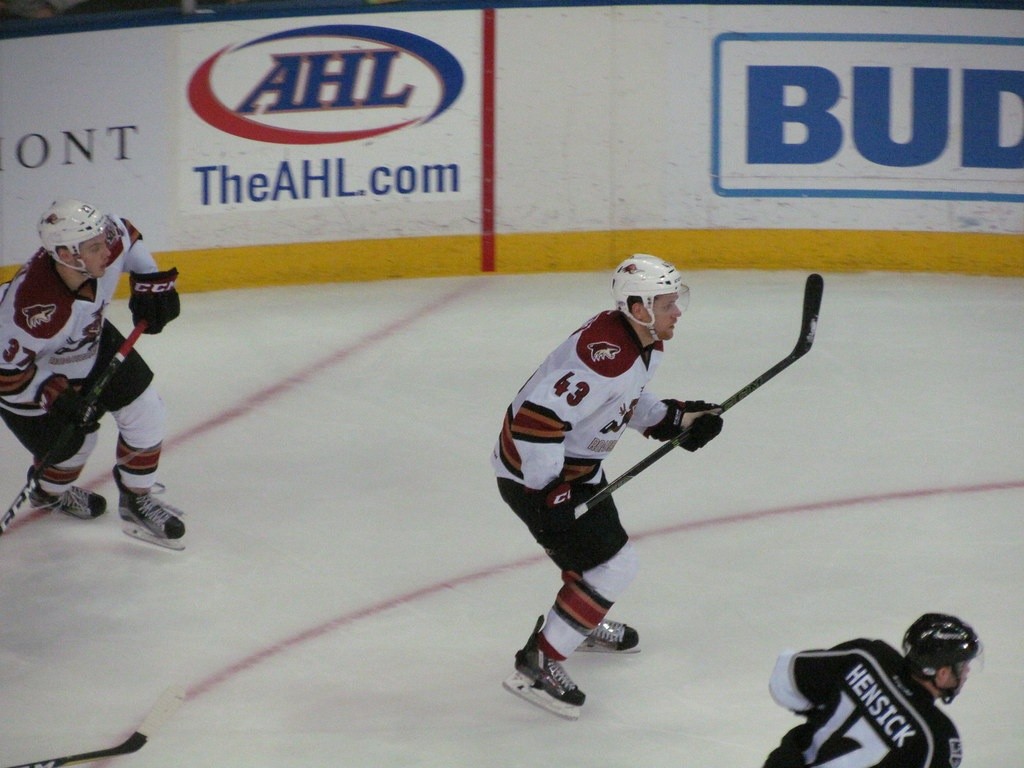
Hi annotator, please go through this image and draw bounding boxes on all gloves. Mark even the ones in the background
[528,476,579,543]
[127,266,181,334]
[36,373,106,435]
[643,399,724,452]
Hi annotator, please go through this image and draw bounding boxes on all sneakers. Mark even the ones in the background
[574,617,640,654]
[27,466,107,519]
[502,614,586,719]
[112,464,187,550]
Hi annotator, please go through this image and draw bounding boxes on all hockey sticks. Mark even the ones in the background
[573,272,827,522]
[0,318,149,539]
[4,684,182,768]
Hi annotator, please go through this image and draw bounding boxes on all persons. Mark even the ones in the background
[760,613,982,767]
[0,199,188,551]
[489,252,724,719]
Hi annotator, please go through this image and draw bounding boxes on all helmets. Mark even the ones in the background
[35,196,107,261]
[902,613,983,683]
[610,253,684,313]
